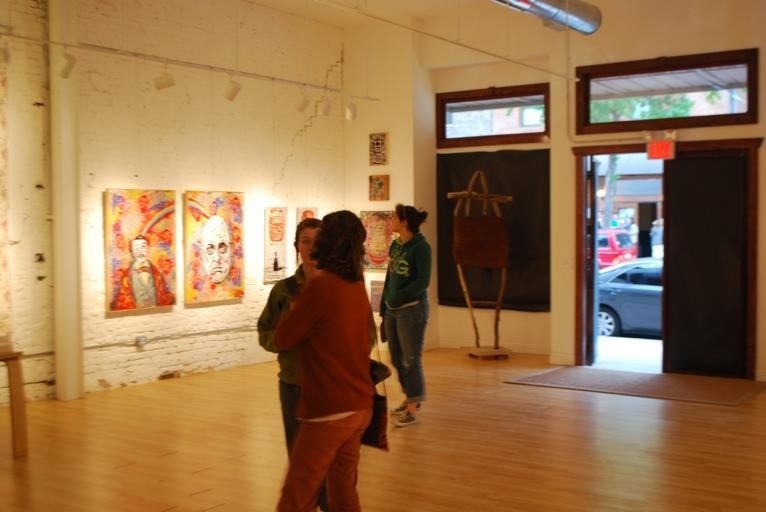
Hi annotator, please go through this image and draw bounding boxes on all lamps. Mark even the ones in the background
[0,32,380,121]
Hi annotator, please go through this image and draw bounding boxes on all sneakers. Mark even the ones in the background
[390,401,423,427]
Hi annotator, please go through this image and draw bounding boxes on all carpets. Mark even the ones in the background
[502,366,766,407]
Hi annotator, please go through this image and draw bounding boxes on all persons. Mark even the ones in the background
[377,200,434,430]
[265,210,378,512]
[255,217,324,512]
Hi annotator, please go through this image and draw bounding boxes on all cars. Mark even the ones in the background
[599,258,664,337]
[584,229,637,271]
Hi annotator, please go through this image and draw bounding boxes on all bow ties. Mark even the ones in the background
[137,267,153,274]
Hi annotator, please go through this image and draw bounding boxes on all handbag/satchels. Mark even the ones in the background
[360,393,391,453]
